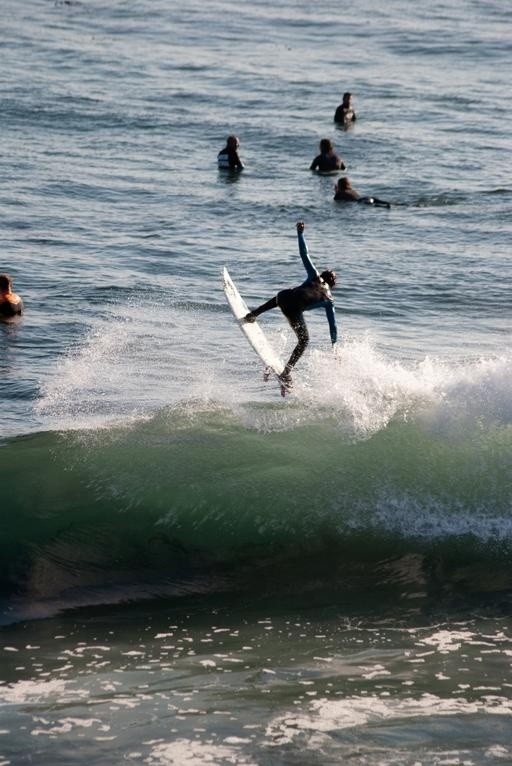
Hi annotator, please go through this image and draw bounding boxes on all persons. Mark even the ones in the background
[244,221,339,390]
[0,274,23,325]
[333,92,356,123]
[332,177,388,205]
[217,135,244,169]
[309,138,346,171]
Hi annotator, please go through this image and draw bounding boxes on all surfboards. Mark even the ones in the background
[219,268,294,396]
